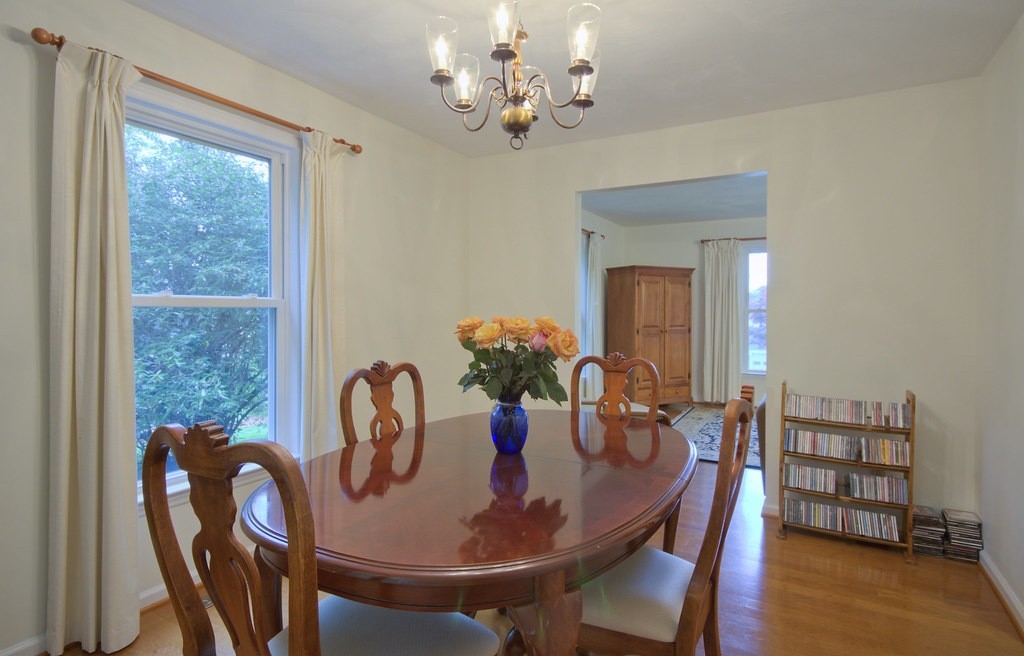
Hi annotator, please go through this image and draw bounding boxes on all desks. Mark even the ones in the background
[240,410,695,655]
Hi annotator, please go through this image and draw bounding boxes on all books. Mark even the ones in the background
[783,392,984,564]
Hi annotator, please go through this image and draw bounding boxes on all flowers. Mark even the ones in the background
[452,315,581,446]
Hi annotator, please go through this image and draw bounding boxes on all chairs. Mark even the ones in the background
[504,384,756,656]
[339,362,426,444]
[143,420,501,655]
[567,352,661,421]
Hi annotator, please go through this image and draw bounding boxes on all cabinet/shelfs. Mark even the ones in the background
[605,264,696,408]
[776,380,917,565]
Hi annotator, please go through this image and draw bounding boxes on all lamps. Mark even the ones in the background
[425,0,602,150]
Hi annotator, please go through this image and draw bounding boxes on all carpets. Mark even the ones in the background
[671,407,761,468]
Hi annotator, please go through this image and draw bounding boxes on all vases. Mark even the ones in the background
[491,401,530,455]
